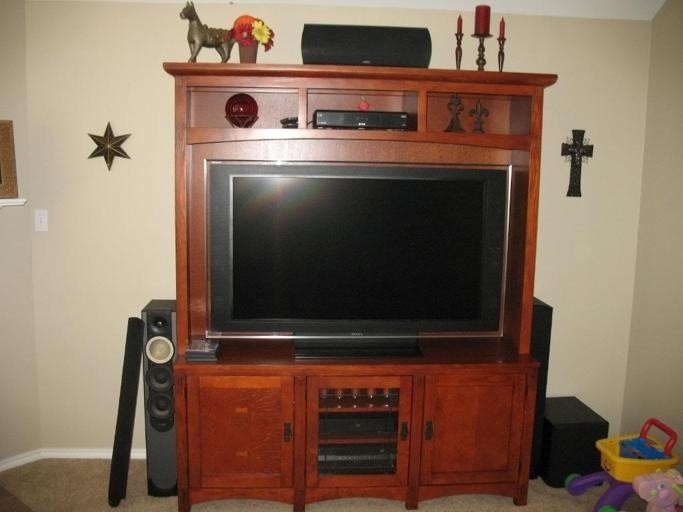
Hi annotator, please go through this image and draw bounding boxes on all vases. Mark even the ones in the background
[238,41,257,63]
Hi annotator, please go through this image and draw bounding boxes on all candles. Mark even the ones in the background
[457,15,462,33]
[499,17,506,37]
[475,5,490,34]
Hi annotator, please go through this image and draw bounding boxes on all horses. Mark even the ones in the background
[179,1,238,64]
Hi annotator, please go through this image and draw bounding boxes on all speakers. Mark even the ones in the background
[142,299,177,497]
[300,23,431,68]
[531,297,553,478]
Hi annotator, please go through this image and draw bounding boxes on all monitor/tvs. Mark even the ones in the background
[204,158,513,358]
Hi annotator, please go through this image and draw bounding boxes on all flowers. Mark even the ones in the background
[225,15,274,51]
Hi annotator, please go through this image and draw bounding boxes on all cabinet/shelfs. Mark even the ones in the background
[162,62,558,512]
